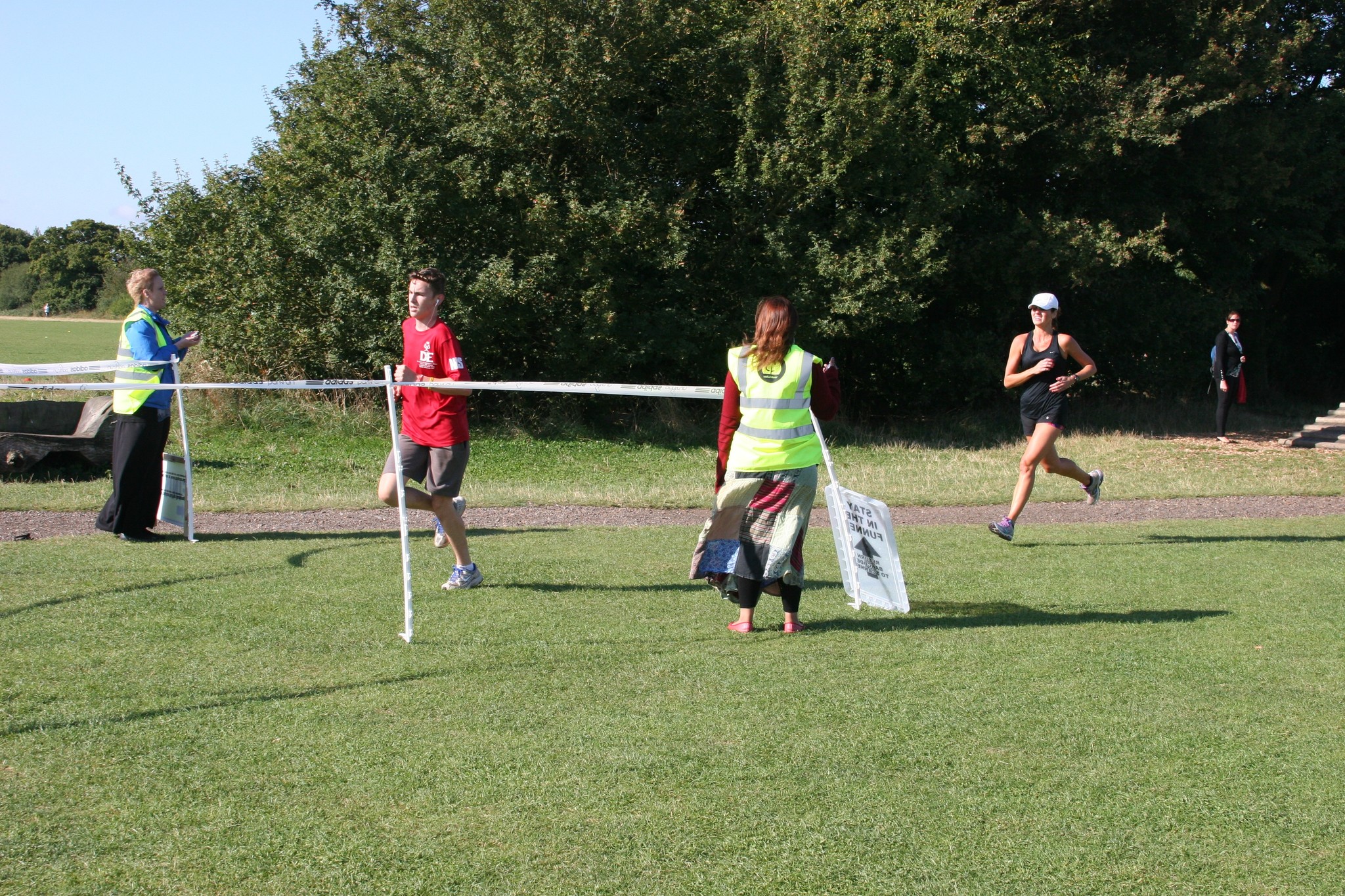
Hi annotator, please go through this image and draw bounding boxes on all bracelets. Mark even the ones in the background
[418,374,423,382]
[428,377,433,390]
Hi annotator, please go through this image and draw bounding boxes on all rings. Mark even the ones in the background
[1223,389,1225,391]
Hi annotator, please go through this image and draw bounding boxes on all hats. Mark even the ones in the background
[1028,293,1059,310]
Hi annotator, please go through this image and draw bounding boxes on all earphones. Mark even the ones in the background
[435,299,439,306]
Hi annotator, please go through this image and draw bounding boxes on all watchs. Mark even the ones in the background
[1071,374,1079,384]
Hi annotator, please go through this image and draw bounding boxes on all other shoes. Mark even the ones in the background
[119,528,165,542]
[784,620,806,634]
[727,622,753,633]
[1216,437,1238,444]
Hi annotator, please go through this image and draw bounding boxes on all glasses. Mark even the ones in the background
[1228,318,1241,322]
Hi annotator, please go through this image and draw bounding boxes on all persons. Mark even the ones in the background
[689,296,841,633]
[1207,311,1246,443]
[377,268,483,590]
[95,268,201,540]
[44,303,50,317]
[989,293,1103,541]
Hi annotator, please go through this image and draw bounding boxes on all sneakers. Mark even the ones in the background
[434,496,465,547]
[1080,470,1104,505]
[989,516,1014,541]
[441,563,484,590]
